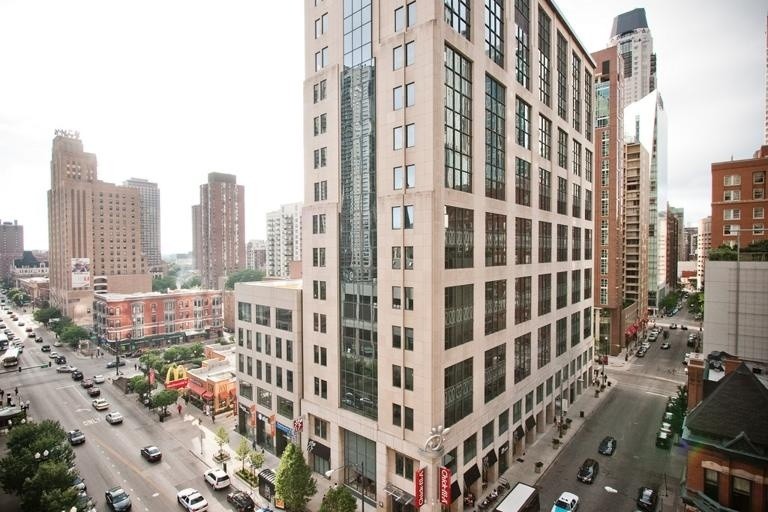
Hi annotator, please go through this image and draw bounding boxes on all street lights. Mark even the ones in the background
[600,343,621,387]
[18,400,29,420]
[20,417,33,425]
[624,331,631,360]
[35,448,50,465]
[558,377,585,438]
[323,461,365,512]
[604,336,608,355]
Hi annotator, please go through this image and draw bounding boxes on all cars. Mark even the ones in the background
[74,475,96,512]
[667,291,702,320]
[177,487,208,512]
[577,458,599,484]
[550,492,579,512]
[68,428,85,446]
[598,436,617,456]
[655,396,680,449]
[257,507,272,512]
[635,322,698,357]
[0,291,66,368]
[56,349,143,425]
[636,486,656,511]
[105,485,132,512]
[140,444,163,462]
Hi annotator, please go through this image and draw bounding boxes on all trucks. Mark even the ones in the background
[492,481,540,512]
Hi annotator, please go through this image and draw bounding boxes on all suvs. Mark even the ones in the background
[204,467,231,491]
[227,490,255,512]
